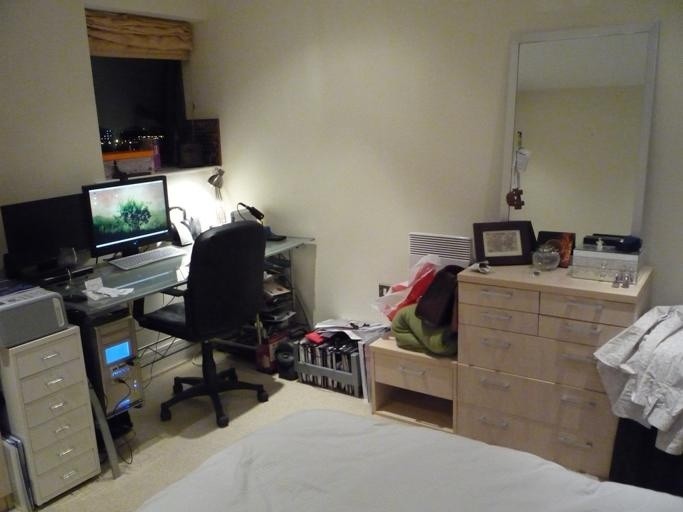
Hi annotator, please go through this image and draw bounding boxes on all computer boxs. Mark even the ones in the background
[66,308,144,419]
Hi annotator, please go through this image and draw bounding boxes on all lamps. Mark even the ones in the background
[208,167,226,188]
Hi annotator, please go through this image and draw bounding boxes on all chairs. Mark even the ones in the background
[137,219,270,428]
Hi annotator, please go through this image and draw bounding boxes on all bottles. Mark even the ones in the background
[532,246,561,271]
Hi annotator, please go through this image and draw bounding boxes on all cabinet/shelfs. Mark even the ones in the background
[457,265,657,480]
[0,322,101,512]
[369,334,457,435]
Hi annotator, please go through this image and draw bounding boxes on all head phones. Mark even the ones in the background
[168,206,191,233]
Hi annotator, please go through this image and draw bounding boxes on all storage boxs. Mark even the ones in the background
[570,247,646,286]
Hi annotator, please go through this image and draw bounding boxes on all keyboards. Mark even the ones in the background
[108,245,186,270]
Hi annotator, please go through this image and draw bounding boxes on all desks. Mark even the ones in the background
[41,235,316,480]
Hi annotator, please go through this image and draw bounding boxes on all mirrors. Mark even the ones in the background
[501,17,661,276]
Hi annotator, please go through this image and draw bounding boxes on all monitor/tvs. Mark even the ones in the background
[81,175,171,261]
[0,193,94,285]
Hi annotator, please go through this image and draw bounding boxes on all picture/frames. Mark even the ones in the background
[473,220,536,268]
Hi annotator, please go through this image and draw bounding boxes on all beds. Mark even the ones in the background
[129,408,683,512]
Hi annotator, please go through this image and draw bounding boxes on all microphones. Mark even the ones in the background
[238,201,264,224]
